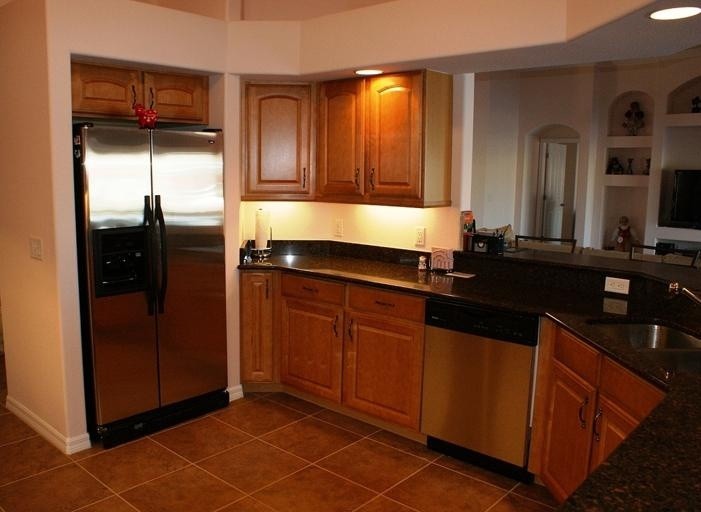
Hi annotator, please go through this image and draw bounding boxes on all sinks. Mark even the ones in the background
[644,349,701,374]
[584,314,701,352]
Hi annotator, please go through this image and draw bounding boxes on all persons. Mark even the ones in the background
[608,215,642,252]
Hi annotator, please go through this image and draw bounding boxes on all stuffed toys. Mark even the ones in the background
[606,155,625,174]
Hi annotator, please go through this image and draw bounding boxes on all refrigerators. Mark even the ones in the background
[70,122,229,449]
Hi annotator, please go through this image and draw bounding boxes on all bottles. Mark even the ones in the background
[418,255,426,270]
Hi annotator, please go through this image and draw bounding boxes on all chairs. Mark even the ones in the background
[515,235,699,265]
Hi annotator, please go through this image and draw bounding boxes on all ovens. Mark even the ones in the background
[419,296,539,469]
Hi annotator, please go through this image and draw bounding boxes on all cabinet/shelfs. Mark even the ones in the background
[239,270,428,448]
[317,69,454,209]
[71,62,209,126]
[241,80,317,200]
[540,322,667,504]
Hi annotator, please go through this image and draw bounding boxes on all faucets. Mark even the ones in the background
[664,280,701,306]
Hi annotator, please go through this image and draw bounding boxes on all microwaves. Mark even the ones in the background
[672,169,701,230]
